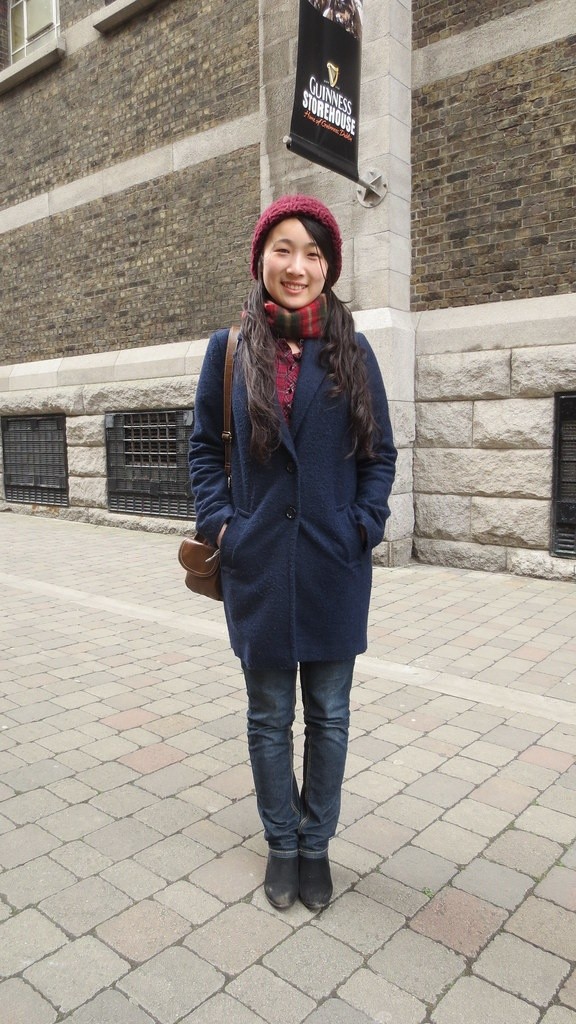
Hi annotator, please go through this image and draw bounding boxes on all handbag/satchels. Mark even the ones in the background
[179,533,224,603]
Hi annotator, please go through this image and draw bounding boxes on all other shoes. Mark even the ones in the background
[299,853,333,909]
[263,853,299,908]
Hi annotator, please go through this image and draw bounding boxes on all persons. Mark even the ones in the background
[186,196,397,912]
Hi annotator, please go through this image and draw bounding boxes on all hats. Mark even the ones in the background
[251,193,341,286]
